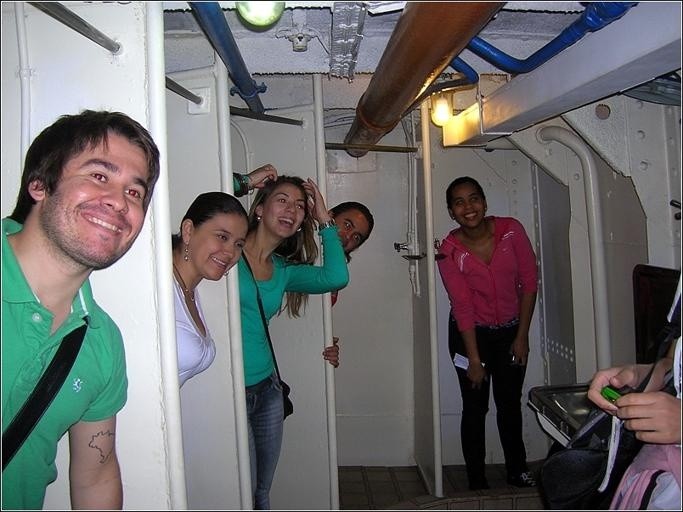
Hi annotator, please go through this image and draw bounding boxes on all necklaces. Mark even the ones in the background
[174,257,196,305]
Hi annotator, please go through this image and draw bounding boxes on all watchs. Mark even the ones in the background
[318,218,335,232]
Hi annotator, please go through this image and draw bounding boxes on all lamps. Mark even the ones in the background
[431,91,453,127]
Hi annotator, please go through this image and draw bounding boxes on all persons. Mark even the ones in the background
[230,164,350,509]
[168,192,249,390]
[322,202,373,368]
[0,112,158,512]
[437,176,541,492]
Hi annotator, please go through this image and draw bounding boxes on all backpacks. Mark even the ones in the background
[544,332,681,512]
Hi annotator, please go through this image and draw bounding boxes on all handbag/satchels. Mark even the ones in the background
[538,271,682,508]
[236,246,294,418]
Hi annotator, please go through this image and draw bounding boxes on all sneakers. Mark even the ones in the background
[509,471,537,487]
